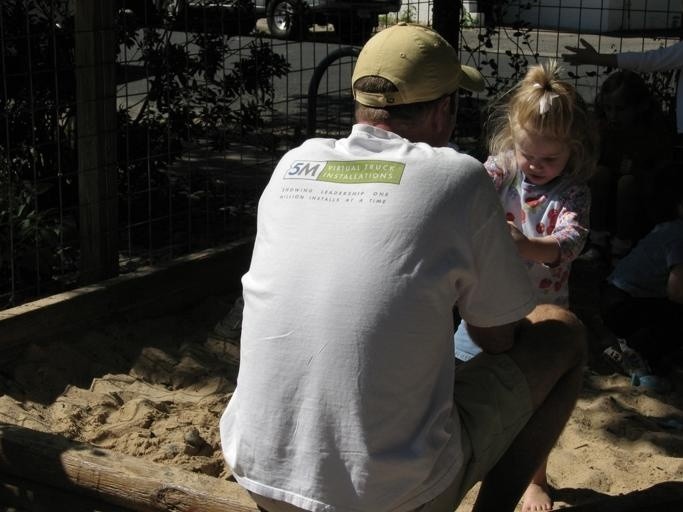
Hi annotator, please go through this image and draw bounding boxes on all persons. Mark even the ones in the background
[445,61,591,510]
[217,16,588,511]
[560,24,683,394]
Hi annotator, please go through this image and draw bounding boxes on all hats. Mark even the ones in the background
[350,21,487,107]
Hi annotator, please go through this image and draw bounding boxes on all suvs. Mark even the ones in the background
[161,1,403,43]
